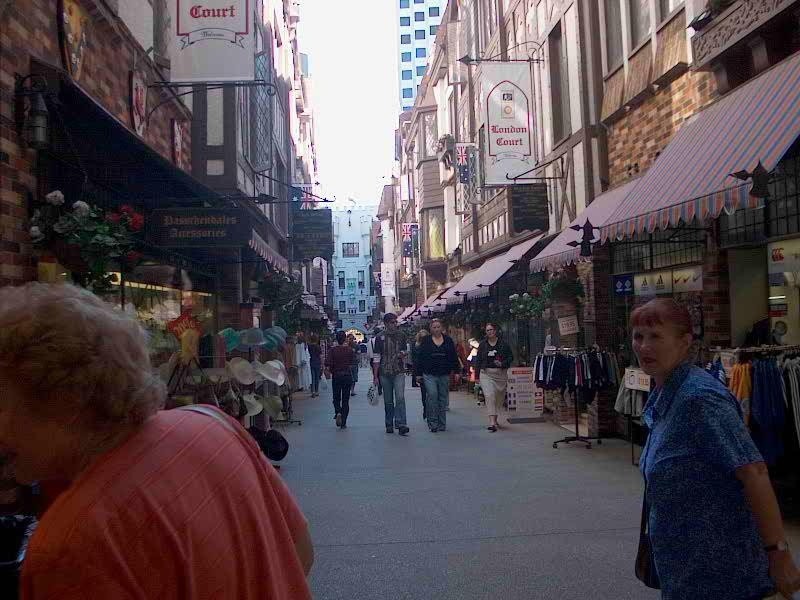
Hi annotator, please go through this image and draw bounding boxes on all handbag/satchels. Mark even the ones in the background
[366,383,379,407]
[635,533,661,589]
[166,356,248,420]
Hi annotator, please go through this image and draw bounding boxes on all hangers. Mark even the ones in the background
[702,344,800,369]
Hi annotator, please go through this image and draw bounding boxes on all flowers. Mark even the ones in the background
[451,277,585,326]
[27,191,144,297]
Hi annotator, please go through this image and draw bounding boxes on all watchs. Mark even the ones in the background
[764,540,790,553]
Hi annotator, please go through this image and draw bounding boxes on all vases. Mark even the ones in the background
[553,291,576,307]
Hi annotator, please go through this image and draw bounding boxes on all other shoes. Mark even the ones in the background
[336,414,342,426]
[386,428,393,433]
[399,426,409,434]
[488,425,496,430]
[341,425,347,429]
[496,424,504,429]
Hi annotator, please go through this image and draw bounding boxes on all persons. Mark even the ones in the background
[627,298,799,600]
[474,323,513,430]
[325,330,354,426]
[344,334,360,396]
[412,329,431,420]
[351,336,373,366]
[0,280,318,600]
[366,327,388,395]
[768,320,789,348]
[307,335,323,399]
[370,312,410,435]
[415,318,462,432]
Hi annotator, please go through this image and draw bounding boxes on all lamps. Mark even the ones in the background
[686,9,714,32]
[12,71,55,150]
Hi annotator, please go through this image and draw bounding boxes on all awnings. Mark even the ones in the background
[27,52,335,331]
[386,53,800,326]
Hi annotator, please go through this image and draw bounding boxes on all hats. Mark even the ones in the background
[250,360,264,389]
[273,359,285,371]
[234,329,257,352]
[260,396,284,420]
[223,328,241,352]
[256,360,285,386]
[262,430,289,461]
[230,357,256,384]
[242,393,263,416]
[263,335,280,353]
[264,326,288,344]
[242,327,266,345]
